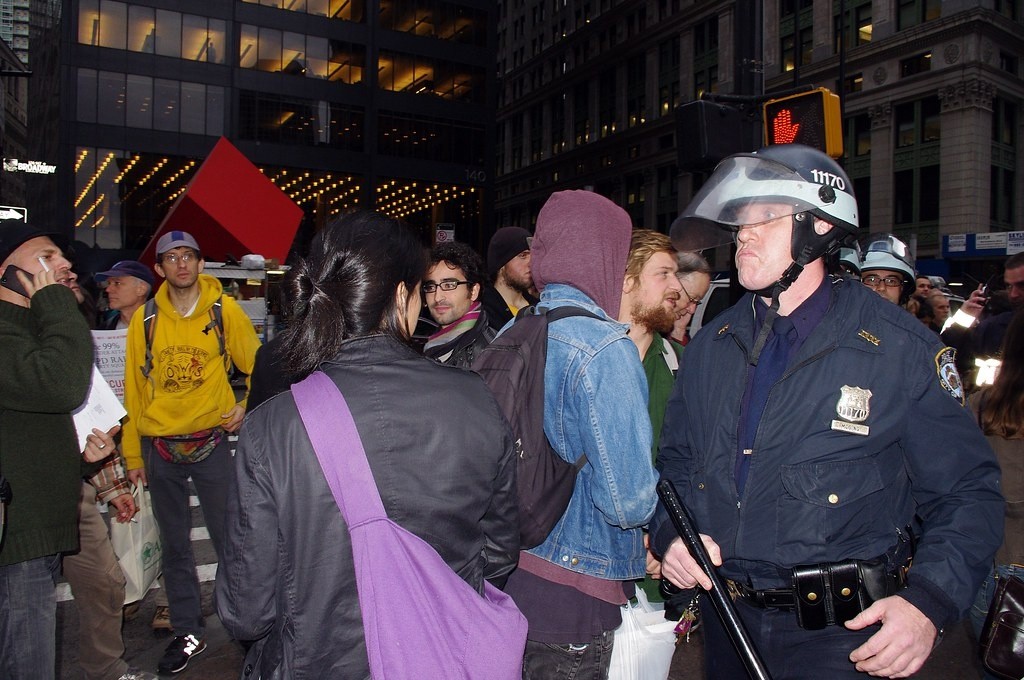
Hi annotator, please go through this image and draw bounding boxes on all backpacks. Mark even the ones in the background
[469,304,609,550]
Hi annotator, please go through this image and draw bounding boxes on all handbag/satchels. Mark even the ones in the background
[975,574,1024,680]
[110,476,163,605]
[607,580,679,680]
[290,370,528,680]
[149,424,227,466]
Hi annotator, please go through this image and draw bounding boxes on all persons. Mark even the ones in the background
[68,270,159,680]
[607,231,685,680]
[494,190,661,680]
[213,212,520,680]
[665,252,710,344]
[123,232,263,673]
[834,233,1024,636]
[649,146,1006,680]
[0,218,120,680]
[423,226,540,368]
[94,261,174,636]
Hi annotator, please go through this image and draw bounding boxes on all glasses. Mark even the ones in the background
[422,280,474,293]
[161,251,197,263]
[862,273,904,287]
[845,268,853,274]
[674,273,702,305]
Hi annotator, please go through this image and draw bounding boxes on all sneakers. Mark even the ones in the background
[157,633,207,674]
[117,664,159,680]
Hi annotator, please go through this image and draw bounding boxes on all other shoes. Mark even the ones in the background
[151,606,174,633]
[122,601,141,622]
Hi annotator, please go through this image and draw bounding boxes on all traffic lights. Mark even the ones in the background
[763,88,843,161]
[676,99,742,173]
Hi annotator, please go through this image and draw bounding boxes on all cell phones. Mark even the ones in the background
[977,286,990,305]
[0,265,36,299]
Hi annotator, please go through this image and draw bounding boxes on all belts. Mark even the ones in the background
[734,556,913,610]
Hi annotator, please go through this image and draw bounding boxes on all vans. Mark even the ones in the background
[205,256,292,385]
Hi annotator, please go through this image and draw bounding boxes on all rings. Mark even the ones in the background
[99,443,106,449]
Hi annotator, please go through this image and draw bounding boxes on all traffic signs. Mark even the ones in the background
[0,205,27,224]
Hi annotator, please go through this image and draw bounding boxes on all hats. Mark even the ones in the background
[94,260,155,287]
[487,226,533,276]
[0,217,69,267]
[155,230,200,262]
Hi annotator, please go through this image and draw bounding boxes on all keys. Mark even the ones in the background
[674,599,698,643]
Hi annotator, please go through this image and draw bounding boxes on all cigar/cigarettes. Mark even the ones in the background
[126,516,137,523]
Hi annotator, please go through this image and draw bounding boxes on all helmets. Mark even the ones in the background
[861,232,916,295]
[833,244,862,277]
[718,142,861,247]
